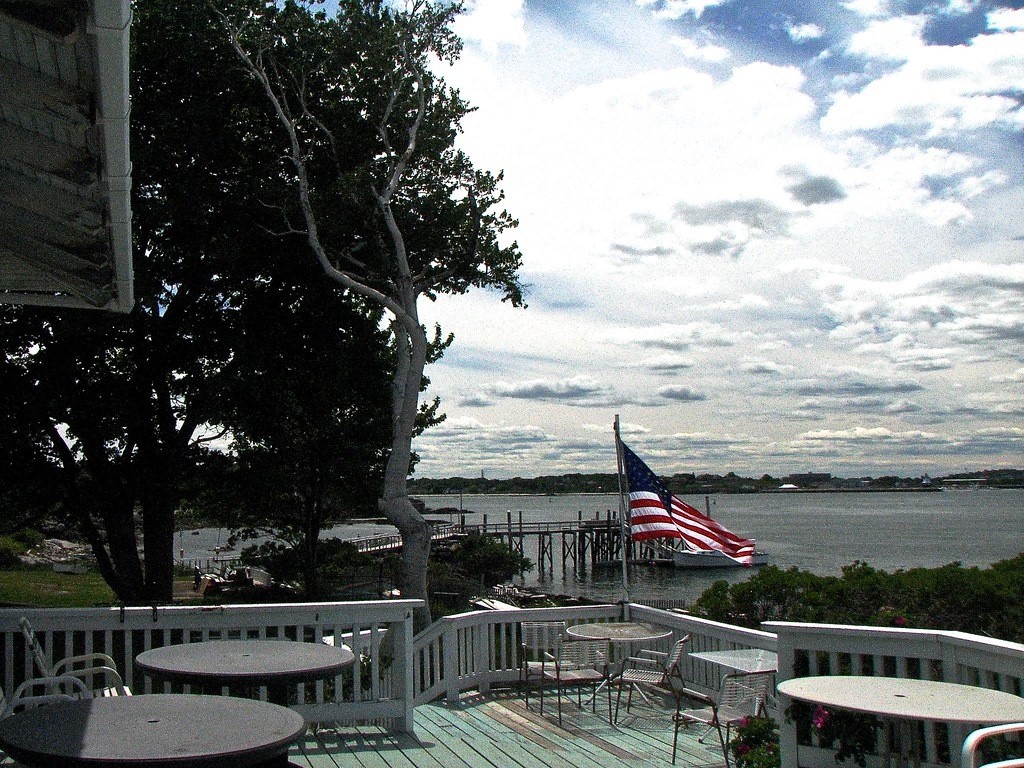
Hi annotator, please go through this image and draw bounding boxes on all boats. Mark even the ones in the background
[673,551,769,566]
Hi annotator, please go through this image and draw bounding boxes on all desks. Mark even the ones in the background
[565,622,674,709]
[136,640,356,708]
[688,649,778,744]
[776,676,1024,768]
[0,693,306,768]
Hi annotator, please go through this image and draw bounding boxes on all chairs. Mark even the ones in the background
[614,633,694,730]
[0,676,92,722]
[518,621,567,710]
[540,638,613,725]
[17,616,132,700]
[671,669,778,768]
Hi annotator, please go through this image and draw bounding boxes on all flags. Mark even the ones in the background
[621,441,756,567]
